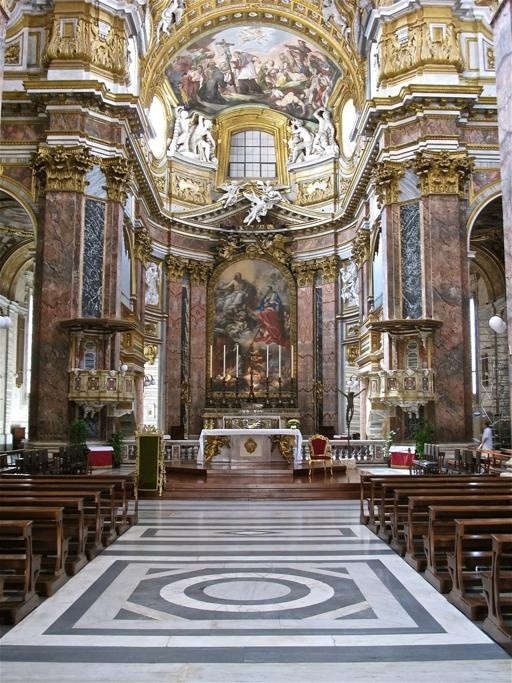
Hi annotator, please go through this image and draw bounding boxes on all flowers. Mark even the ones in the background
[285,418,300,427]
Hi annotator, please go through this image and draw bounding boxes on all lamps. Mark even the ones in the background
[489,306,507,333]
[347,374,363,387]
[0,305,12,328]
[118,358,128,373]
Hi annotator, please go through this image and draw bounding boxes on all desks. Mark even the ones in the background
[195,428,303,477]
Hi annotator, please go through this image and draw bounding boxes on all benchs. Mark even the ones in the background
[358,472,512,657]
[0,472,131,624]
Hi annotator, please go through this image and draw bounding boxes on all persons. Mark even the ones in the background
[282,105,343,163]
[173,31,336,111]
[218,174,287,224]
[214,269,291,377]
[477,420,493,458]
[154,2,178,42]
[335,258,360,308]
[172,105,218,162]
[333,385,366,428]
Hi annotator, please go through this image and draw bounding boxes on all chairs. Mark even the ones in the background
[307,433,334,476]
[0,444,92,475]
[408,448,498,474]
